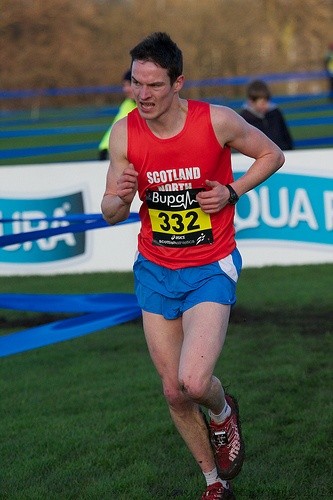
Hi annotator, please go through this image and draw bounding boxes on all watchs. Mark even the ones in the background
[224,184,239,207]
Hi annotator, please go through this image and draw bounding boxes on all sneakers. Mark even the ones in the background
[201,480,231,500]
[209,392,245,480]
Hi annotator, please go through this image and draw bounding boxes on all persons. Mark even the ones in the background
[323,42,333,103]
[98,67,139,162]
[237,77,294,152]
[100,32,285,500]
